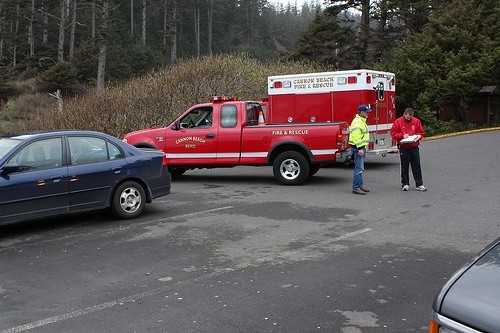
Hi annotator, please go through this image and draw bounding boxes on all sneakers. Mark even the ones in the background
[415,185,427,191]
[402,184,410,191]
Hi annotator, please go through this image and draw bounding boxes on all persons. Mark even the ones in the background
[390,108,428,191]
[347,105,372,195]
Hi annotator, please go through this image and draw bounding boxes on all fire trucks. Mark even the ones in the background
[248,69,400,158]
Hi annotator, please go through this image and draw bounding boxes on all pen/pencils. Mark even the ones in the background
[403,132,408,138]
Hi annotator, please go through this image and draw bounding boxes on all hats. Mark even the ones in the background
[358,105,372,112]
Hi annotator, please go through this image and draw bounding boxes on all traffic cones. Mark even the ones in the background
[258,111,266,126]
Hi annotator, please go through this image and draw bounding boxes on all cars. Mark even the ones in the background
[430,237,500,333]
[0,129,171,225]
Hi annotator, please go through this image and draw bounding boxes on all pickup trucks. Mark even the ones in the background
[120,95,349,186]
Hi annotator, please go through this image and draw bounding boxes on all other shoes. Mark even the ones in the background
[352,187,369,195]
